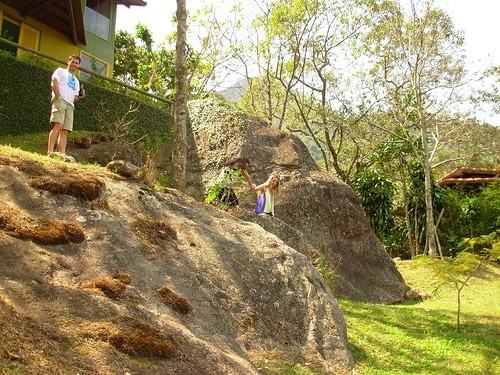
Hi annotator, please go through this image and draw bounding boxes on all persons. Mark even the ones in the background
[47,55,85,155]
[212,157,256,207]
[250,174,280,218]
